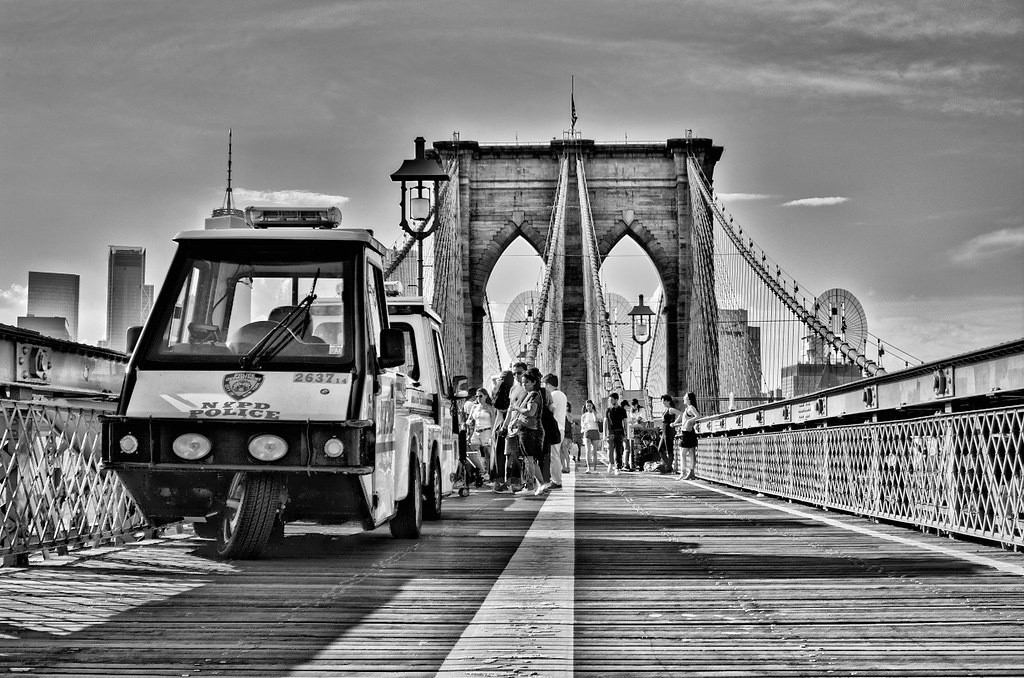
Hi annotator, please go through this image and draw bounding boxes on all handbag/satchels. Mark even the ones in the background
[542,406,562,447]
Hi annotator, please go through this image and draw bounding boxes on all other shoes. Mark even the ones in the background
[632,465,636,469]
[608,463,613,473]
[548,482,562,490]
[585,469,591,474]
[562,468,570,473]
[594,471,598,475]
[625,466,630,469]
[615,469,621,475]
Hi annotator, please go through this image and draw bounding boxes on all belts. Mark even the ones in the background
[476,427,491,433]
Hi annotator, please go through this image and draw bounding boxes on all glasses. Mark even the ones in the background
[475,395,482,398]
[513,372,523,376]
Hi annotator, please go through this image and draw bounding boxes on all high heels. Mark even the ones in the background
[683,469,696,480]
[675,470,687,481]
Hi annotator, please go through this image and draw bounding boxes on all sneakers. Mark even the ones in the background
[535,484,548,495]
[515,484,536,495]
[494,484,513,493]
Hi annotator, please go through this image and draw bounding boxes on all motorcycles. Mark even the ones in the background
[99,202,437,558]
[303,283,472,498]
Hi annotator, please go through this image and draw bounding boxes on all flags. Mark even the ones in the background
[571,93,578,128]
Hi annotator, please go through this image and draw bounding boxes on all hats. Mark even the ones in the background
[522,368,542,381]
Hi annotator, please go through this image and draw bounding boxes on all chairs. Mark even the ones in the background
[268,306,325,344]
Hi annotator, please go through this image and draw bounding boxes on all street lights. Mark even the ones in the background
[626,296,654,400]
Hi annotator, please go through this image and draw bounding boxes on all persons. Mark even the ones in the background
[462,362,583,496]
[580,393,700,480]
[458,383,468,397]
